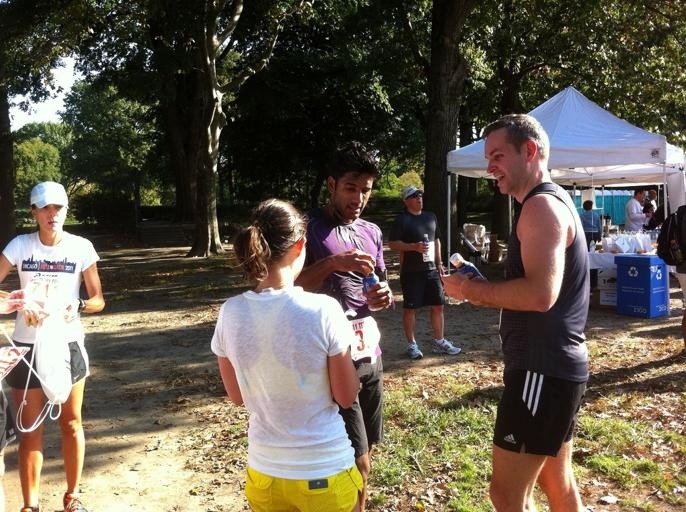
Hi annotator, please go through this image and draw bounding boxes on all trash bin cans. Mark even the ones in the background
[614,254,671,319]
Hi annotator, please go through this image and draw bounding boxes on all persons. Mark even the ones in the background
[389,186,461,359]
[295,140,395,512]
[441,113,590,511]
[626,189,686,356]
[211,198,363,512]
[580,200,602,245]
[1,288,30,315]
[1,182,105,511]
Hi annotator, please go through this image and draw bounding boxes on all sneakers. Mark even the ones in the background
[431,339,462,355]
[61,492,87,511]
[407,343,423,358]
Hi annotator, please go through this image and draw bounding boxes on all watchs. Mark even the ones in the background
[78,298,87,313]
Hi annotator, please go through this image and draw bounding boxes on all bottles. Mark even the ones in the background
[589,239,596,254]
[362,269,386,312]
[449,252,488,283]
[422,232,431,264]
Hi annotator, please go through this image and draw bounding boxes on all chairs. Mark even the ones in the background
[465,224,508,264]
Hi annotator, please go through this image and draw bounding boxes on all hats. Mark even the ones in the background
[28,181,69,210]
[403,186,423,200]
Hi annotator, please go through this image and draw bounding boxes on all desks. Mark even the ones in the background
[588,249,658,309]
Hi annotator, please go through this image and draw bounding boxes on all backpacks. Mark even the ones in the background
[0,298,71,433]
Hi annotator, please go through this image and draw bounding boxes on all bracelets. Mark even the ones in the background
[436,262,442,266]
[460,278,467,300]
